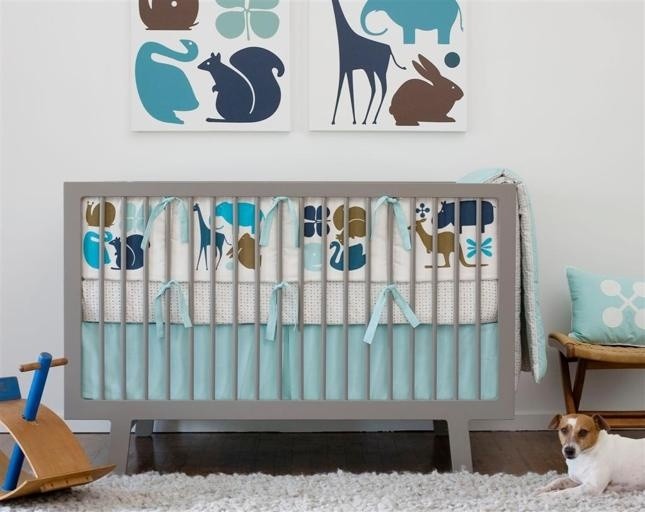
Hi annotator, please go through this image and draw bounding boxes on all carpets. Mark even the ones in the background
[1,470,644,512]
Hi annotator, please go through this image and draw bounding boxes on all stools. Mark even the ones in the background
[547,332,645,433]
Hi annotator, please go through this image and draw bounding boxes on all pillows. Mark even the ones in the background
[565,267,644,346]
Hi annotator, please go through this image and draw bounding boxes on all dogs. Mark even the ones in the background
[536,413,645,499]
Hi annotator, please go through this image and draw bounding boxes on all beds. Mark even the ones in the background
[64,182,516,473]
